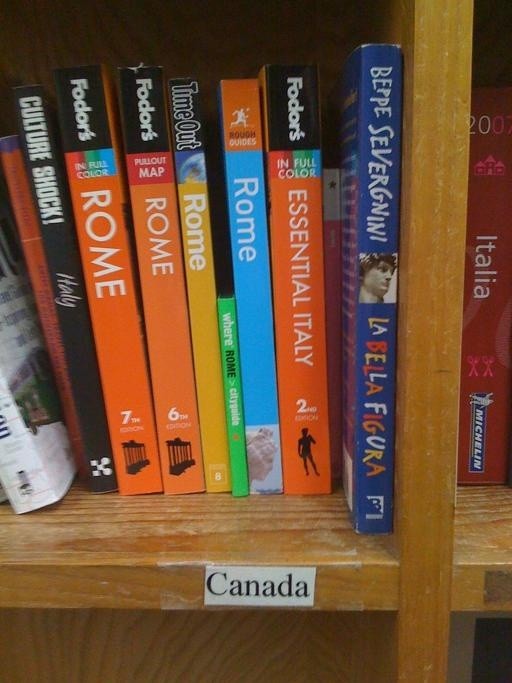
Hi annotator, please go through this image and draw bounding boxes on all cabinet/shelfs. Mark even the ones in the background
[0,0,510,683]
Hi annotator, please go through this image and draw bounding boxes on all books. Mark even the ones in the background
[1,42,406,534]
[455,85,511,487]
